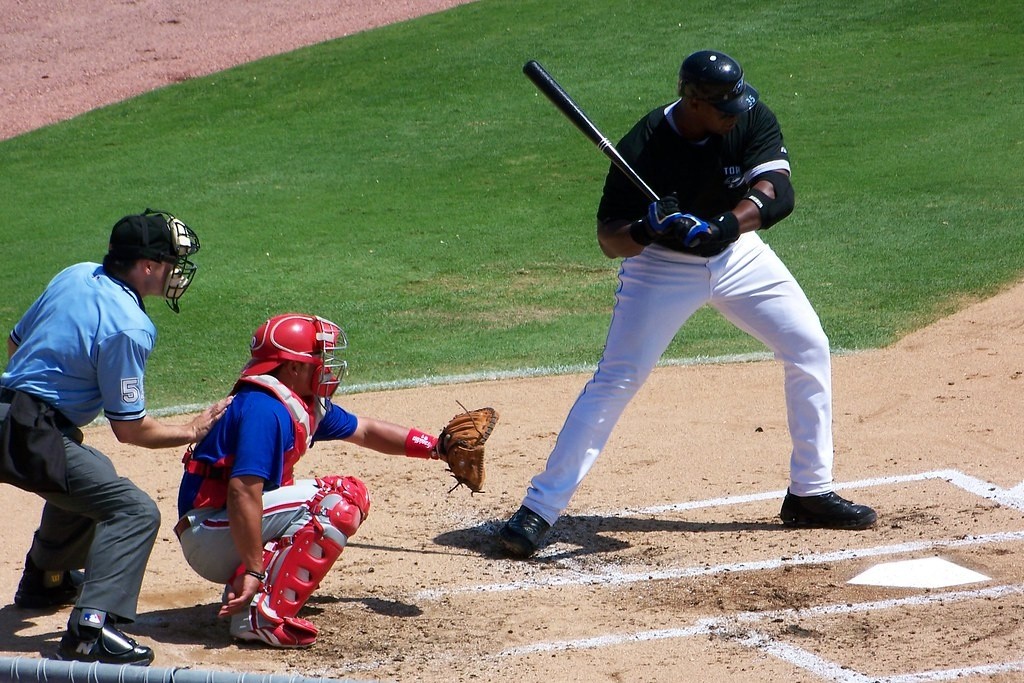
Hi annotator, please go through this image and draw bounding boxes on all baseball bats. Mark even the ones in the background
[521,59,702,249]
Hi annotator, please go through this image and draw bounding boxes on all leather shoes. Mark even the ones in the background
[56,608,154,666]
[14,570,85,608]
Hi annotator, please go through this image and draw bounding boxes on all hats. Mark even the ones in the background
[108,216,177,264]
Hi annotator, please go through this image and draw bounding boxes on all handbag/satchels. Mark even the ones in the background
[0,391,70,495]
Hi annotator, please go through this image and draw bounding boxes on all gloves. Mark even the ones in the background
[630,191,682,246]
[663,212,739,257]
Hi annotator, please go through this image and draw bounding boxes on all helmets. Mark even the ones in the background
[677,51,759,114]
[240,314,322,375]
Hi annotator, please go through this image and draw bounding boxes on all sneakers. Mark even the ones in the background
[780,487,877,529]
[498,504,550,560]
[231,606,317,649]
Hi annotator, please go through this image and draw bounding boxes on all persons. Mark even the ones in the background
[499,51,879,557]
[173,313,496,645]
[0,209,236,666]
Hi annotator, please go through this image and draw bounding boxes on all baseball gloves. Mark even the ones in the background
[438,400,500,497]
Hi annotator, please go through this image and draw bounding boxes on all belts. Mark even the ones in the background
[0,387,84,444]
[173,517,191,539]
